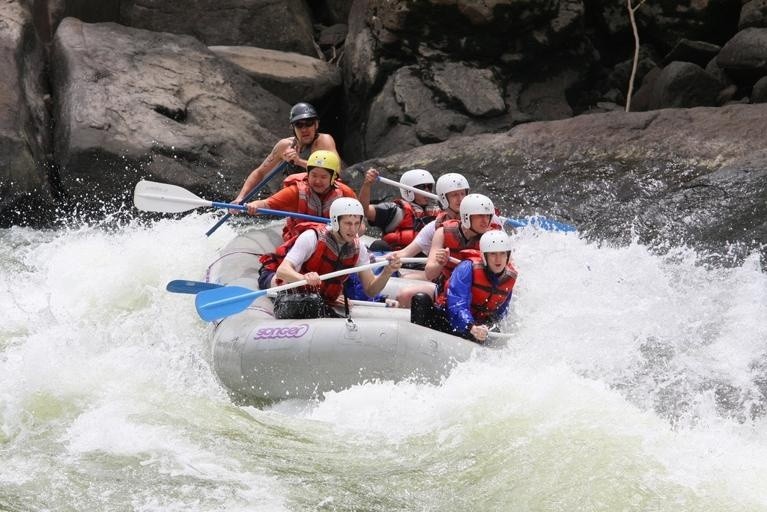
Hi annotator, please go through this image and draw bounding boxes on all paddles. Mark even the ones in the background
[195,259,391,322]
[133,179,331,224]
[376,176,578,236]
[176,159,294,263]
[166,279,395,308]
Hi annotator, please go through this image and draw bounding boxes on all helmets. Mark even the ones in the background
[479,230,513,267]
[307,150,341,180]
[288,102,320,124]
[460,193,495,230]
[329,196,364,232]
[399,169,435,202]
[435,172,470,210]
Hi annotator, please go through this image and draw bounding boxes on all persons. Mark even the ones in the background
[244,149,366,242]
[334,261,401,309]
[410,229,518,346]
[383,173,506,257]
[258,198,402,308]
[424,193,506,286]
[228,102,341,214]
[359,168,439,251]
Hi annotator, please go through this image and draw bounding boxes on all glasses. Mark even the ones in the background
[294,120,314,129]
[413,184,433,190]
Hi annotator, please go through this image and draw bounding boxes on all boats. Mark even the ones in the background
[211,222,493,400]
